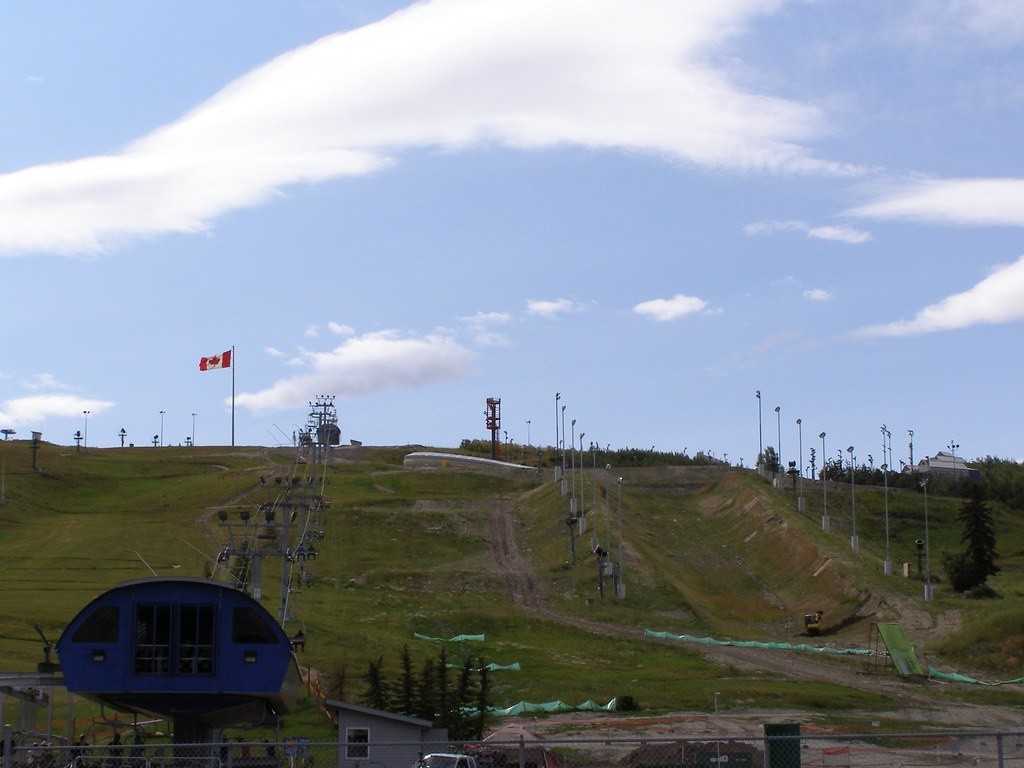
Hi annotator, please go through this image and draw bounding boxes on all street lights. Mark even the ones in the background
[589,445,598,537]
[192,413,197,446]
[525,420,531,444]
[555,391,561,467]
[578,433,586,518]
[83,410,90,448]
[756,390,762,463]
[818,432,828,516]
[561,404,567,481]
[159,410,166,448]
[847,446,857,536]
[795,418,802,497]
[714,691,721,768]
[880,463,890,561]
[604,464,613,560]
[571,419,591,498]
[774,406,782,479]
[918,477,931,585]
[616,474,624,584]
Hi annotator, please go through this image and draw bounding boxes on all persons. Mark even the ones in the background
[26,740,56,768]
[108,734,123,768]
[807,610,823,633]
[265,739,275,756]
[70,734,93,768]
[126,735,146,768]
[218,735,232,762]
[234,735,251,757]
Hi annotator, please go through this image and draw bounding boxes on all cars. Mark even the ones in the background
[411,752,478,768]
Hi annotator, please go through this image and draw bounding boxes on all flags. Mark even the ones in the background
[199,350,231,371]
